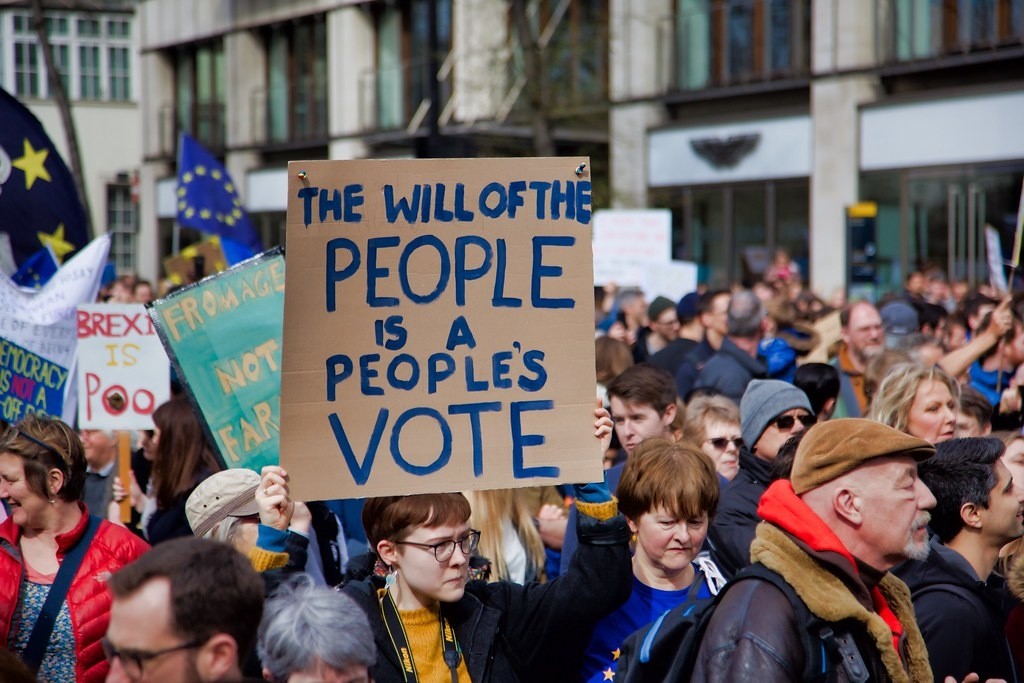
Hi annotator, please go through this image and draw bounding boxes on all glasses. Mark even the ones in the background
[0,424,67,471]
[774,415,818,431]
[394,529,481,562]
[656,319,679,326]
[143,429,160,440]
[706,437,744,451]
[101,633,213,681]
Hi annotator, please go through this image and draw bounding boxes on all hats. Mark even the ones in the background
[677,292,703,317]
[880,303,918,336]
[647,296,676,322]
[790,416,937,495]
[185,467,259,539]
[740,379,815,452]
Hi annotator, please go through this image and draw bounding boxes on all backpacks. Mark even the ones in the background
[615,562,822,683]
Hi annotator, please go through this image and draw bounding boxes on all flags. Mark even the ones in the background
[1,83,259,422]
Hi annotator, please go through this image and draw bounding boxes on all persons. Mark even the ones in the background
[0,248,1024,683]
